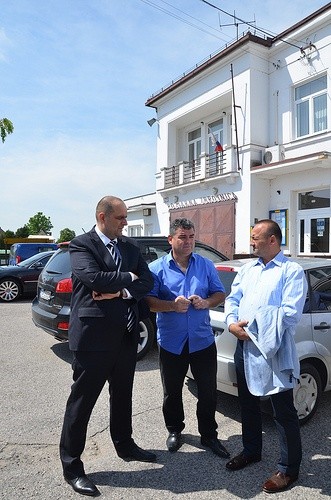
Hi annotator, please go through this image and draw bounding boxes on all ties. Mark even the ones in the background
[107,241,136,332]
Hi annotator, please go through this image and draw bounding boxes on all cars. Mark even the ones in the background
[182,252,331,426]
[0,250,57,303]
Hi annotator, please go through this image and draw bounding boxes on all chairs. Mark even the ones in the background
[147,252,158,260]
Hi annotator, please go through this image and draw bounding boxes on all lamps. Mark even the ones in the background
[148,118,158,126]
[143,208,151,216]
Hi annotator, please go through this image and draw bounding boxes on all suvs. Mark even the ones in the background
[31,235,230,367]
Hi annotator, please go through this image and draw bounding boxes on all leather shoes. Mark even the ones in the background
[263,471,297,492]
[226,456,251,470]
[127,445,157,462]
[200,435,232,457]
[64,474,98,495]
[166,432,181,452]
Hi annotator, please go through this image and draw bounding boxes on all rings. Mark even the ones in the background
[198,303,199,305]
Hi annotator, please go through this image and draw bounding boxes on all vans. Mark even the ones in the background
[9,243,58,266]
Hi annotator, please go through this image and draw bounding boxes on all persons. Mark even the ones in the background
[147,217,229,459]
[223,220,301,492]
[60,196,157,495]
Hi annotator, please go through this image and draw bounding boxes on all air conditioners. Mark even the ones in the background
[261,145,284,165]
